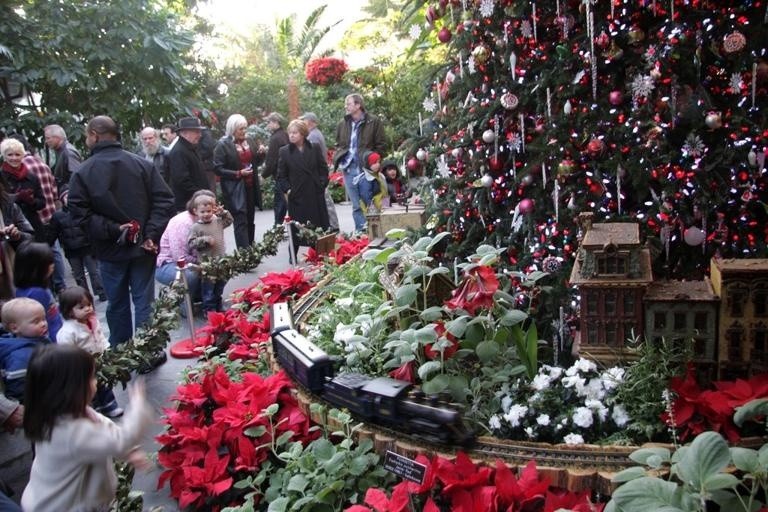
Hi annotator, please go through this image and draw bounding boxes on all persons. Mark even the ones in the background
[136,111,340,320]
[379,160,412,204]
[0,94,174,511]
[335,93,390,235]
[356,149,388,219]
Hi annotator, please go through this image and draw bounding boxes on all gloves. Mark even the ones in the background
[15,186,32,202]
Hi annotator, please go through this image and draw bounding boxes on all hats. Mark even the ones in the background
[173,116,206,131]
[368,153,380,165]
[299,113,317,121]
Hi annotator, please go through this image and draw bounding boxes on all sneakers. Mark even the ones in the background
[108,407,122,417]
[143,351,166,373]
